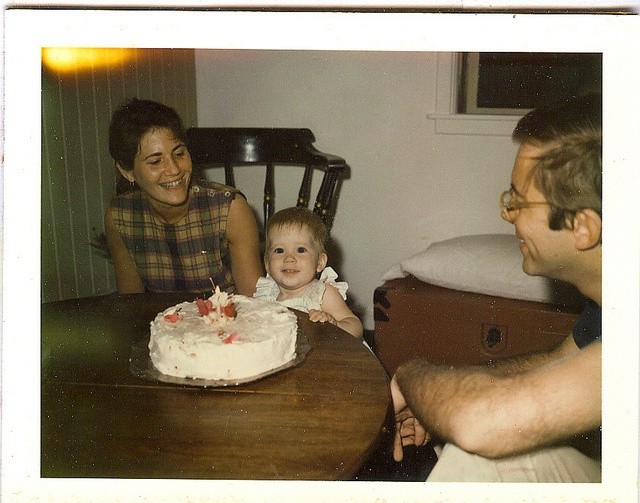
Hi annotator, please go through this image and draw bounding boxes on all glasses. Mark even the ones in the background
[499,187,554,224]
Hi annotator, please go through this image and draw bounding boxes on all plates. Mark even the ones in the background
[125,321,312,388]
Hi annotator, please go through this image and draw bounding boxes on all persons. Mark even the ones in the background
[105,98,265,297]
[389,92,602,482]
[255,206,365,338]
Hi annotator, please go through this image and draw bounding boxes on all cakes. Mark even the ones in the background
[149,286,296,379]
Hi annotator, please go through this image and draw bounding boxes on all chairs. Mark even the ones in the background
[113,125,352,267]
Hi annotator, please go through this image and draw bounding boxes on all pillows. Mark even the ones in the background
[397,229,569,305]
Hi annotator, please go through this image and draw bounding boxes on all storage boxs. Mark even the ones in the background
[373,277,585,379]
